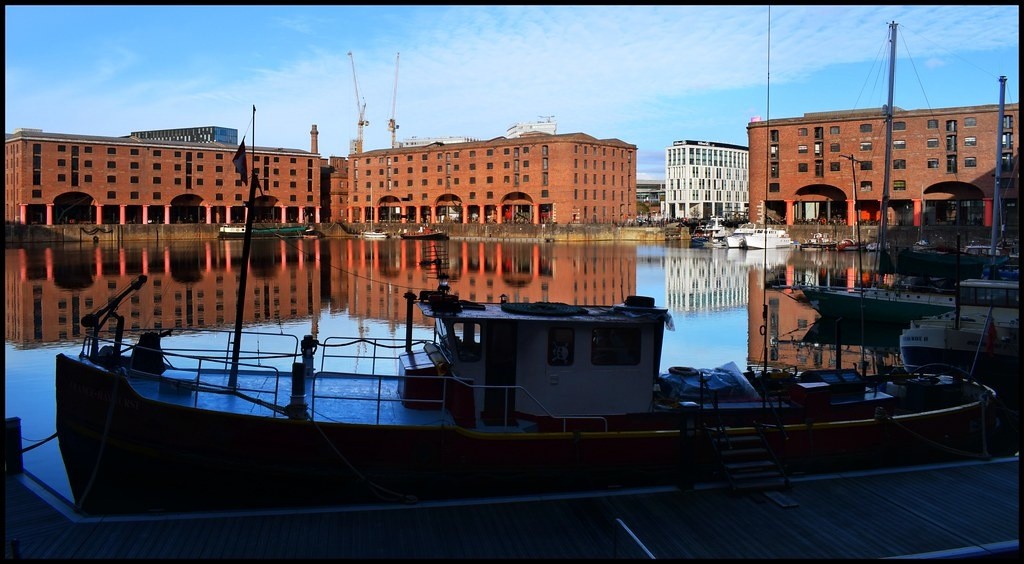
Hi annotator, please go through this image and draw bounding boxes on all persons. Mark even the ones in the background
[612,215,681,228]
[766,215,846,225]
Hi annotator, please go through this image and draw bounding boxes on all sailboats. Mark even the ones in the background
[213,104,307,239]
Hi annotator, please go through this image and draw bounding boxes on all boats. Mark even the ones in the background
[56,172,1005,516]
[355,182,390,239]
[688,21,1020,390]
[399,231,448,240]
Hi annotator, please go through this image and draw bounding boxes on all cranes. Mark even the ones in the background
[388,49,401,149]
[345,50,369,156]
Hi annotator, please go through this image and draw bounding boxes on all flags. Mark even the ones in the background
[232,139,248,186]
